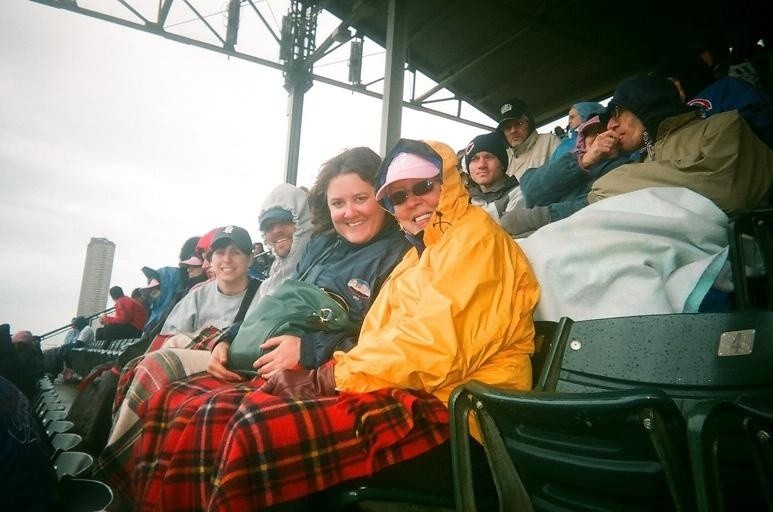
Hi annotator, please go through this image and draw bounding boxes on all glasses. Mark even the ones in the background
[610,104,624,120]
[388,178,433,206]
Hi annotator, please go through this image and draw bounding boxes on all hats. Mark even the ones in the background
[141,279,159,289]
[497,101,521,125]
[179,256,202,266]
[465,135,508,173]
[259,209,292,230]
[211,225,252,256]
[375,151,439,202]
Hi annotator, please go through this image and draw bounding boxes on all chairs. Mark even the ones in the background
[36,338,141,512]
[447,312,772,512]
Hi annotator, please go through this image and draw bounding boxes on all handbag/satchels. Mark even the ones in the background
[226,278,349,376]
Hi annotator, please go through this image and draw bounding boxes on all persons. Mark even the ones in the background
[0,323,44,401]
[457,47,773,316]
[63,139,543,512]
